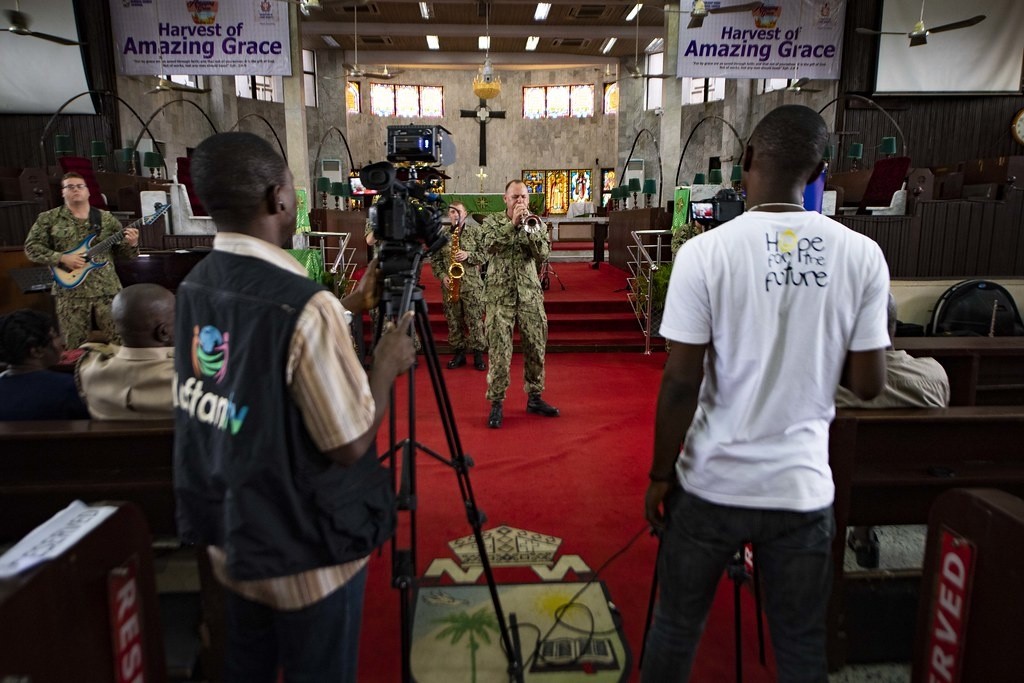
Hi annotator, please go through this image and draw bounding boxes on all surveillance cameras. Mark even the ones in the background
[483,66,493,83]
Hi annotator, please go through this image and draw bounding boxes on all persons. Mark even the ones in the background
[173,131,423,683]
[364,203,423,351]
[479,179,561,428]
[632,102,891,683]
[832,294,951,412]
[24,170,140,352]
[0,283,175,421]
[430,201,488,370]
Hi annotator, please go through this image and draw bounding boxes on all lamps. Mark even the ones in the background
[611,178,656,211]
[693,165,743,190]
[55,134,165,179]
[822,136,896,174]
[317,177,361,212]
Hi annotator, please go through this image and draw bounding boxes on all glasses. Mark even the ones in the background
[62,184,88,190]
[39,323,60,345]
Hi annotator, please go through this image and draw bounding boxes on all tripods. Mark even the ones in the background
[370,235,527,683]
[540,260,566,292]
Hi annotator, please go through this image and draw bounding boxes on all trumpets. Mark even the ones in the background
[520,207,542,234]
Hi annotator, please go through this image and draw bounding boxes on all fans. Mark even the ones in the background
[753,0,823,97]
[0,0,92,54]
[602,0,676,84]
[141,0,213,96]
[855,0,987,47]
[654,0,764,29]
[319,3,405,80]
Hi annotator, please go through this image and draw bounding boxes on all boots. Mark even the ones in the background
[488,400,503,428]
[525,394,559,417]
[473,349,486,370]
[447,349,466,370]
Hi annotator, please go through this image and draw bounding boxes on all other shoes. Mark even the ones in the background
[848,529,880,568]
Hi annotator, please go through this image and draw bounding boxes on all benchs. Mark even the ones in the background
[0,400,206,544]
[830,404,1024,672]
[894,337,1024,406]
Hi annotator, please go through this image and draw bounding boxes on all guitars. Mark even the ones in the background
[49,204,172,288]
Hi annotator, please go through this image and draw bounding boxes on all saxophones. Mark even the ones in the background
[447,219,464,303]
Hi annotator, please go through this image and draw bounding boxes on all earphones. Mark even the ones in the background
[279,200,285,211]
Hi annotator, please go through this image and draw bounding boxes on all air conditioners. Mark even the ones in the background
[136,139,167,177]
[320,158,343,214]
[625,158,645,210]
